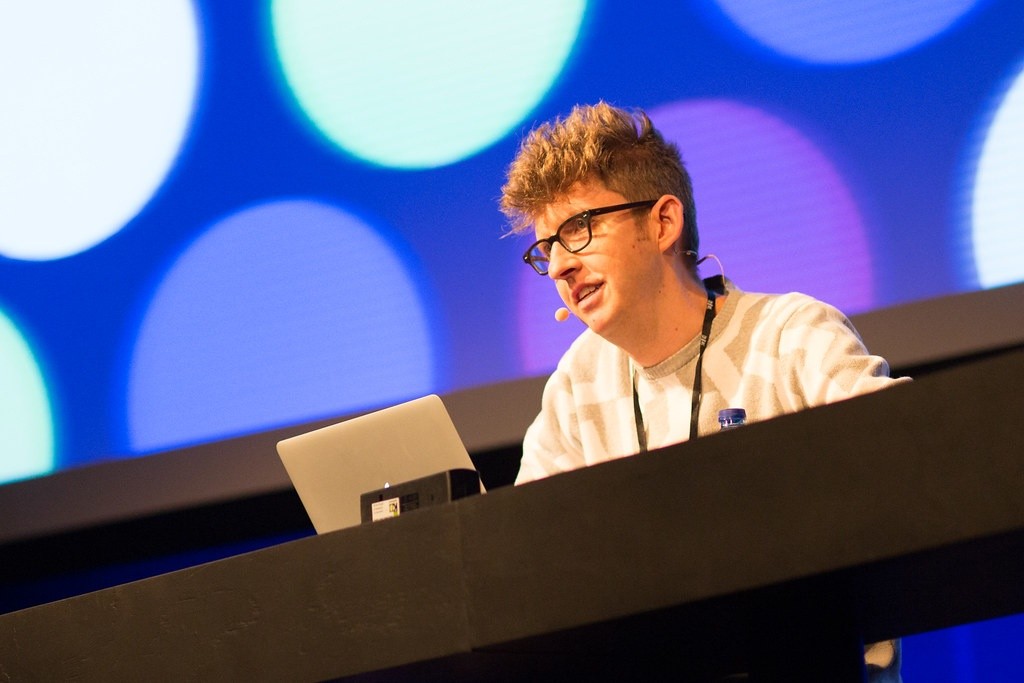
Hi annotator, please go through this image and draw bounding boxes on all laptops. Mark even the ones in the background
[275,395,487,536]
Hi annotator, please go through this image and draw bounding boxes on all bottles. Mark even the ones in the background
[718,409,746,432]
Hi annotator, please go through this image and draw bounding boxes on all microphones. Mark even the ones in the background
[554,307,572,321]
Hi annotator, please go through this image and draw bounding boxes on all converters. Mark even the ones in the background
[358,470,480,525]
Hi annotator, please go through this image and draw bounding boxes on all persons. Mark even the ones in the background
[500,105,912,683]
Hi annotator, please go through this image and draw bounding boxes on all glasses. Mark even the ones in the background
[523,199,661,275]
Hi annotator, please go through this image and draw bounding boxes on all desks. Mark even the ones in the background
[0,349,1024,683]
